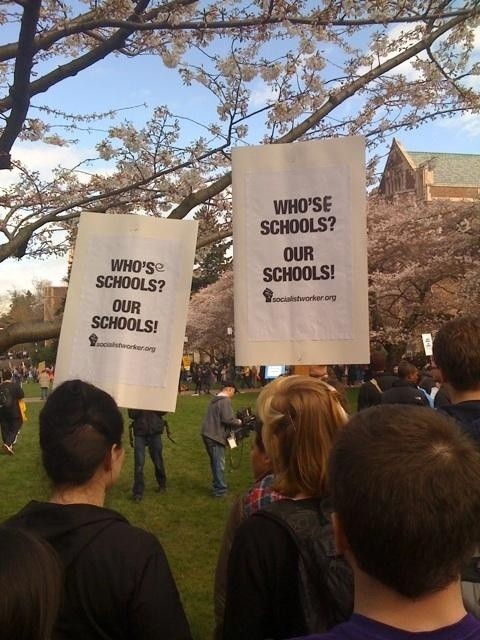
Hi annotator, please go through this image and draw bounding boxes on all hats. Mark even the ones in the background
[224,382,241,393]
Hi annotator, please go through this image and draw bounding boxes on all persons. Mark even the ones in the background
[178,357,265,395]
[224,374,354,640]
[200,382,243,496]
[291,405,480,640]
[1,349,54,454]
[0,379,190,640]
[432,317,480,621]
[287,350,451,414]
[127,408,177,501]
[213,419,287,640]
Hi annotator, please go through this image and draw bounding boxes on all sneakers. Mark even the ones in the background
[2,443,14,455]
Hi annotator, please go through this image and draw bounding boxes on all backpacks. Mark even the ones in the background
[0,382,13,411]
[252,499,354,634]
